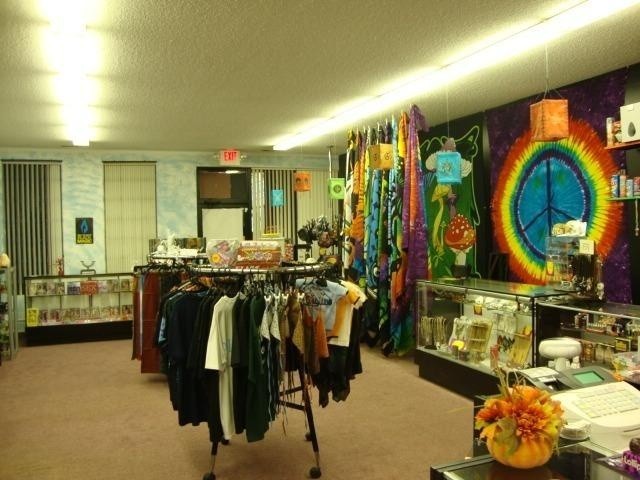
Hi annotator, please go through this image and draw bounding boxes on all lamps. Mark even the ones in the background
[436,88,462,185]
[269,188,284,208]
[368,110,394,170]
[294,144,312,191]
[529,44,570,142]
[327,131,345,200]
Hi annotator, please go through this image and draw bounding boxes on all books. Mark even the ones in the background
[26,278,134,327]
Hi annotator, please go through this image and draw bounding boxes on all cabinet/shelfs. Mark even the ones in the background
[23,272,134,347]
[536,297,640,368]
[604,139,640,202]
[415,278,581,402]
[0,265,20,361]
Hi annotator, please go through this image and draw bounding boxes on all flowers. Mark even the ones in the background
[447,366,567,458]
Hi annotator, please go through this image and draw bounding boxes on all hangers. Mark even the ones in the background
[134,255,337,306]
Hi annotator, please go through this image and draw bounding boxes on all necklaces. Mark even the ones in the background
[420,315,493,361]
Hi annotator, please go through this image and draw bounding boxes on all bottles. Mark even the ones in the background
[584,343,616,366]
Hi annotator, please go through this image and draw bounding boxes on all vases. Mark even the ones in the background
[486,435,554,469]
